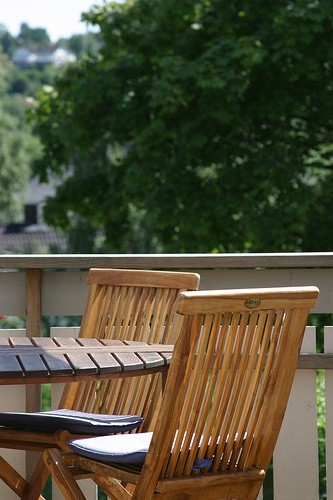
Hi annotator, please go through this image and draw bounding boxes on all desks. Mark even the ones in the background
[1,323,174,500]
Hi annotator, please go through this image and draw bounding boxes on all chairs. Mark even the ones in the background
[1,262,205,499]
[42,281,323,499]
